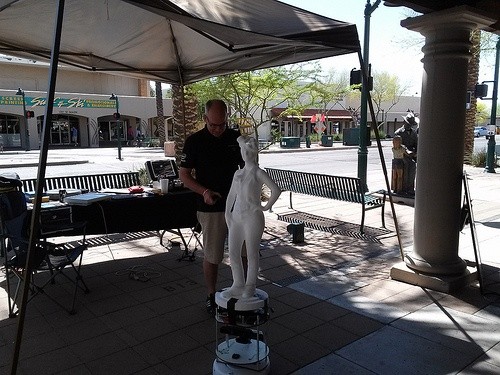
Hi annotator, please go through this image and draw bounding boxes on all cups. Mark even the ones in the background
[160,179,168,194]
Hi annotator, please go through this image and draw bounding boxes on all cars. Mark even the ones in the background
[473,126,488,138]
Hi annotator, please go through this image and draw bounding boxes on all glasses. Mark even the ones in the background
[206,116,227,129]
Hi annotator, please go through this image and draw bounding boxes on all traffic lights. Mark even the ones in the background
[26,110,32,117]
[114,113,120,120]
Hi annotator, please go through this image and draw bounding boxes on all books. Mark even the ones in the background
[65,193,111,206]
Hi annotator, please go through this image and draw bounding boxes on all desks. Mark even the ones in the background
[22,182,195,233]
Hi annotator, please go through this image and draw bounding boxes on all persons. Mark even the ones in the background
[71,126,78,148]
[221,136,281,299]
[179,99,248,319]
[135,128,140,138]
[392,110,418,198]
[126,126,134,147]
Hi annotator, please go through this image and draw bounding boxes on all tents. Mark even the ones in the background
[0,0,406,375]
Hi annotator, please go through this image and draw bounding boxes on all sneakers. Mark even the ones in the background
[207,291,218,314]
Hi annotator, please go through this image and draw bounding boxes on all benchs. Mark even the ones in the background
[263,166,389,233]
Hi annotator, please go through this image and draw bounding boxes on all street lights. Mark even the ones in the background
[15,88,31,151]
[109,92,121,159]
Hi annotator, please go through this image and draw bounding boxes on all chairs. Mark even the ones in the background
[155,191,203,254]
[1,217,92,318]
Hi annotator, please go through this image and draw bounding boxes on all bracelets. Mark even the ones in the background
[202,188,208,196]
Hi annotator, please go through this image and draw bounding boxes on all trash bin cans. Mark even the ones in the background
[322,135,333,147]
